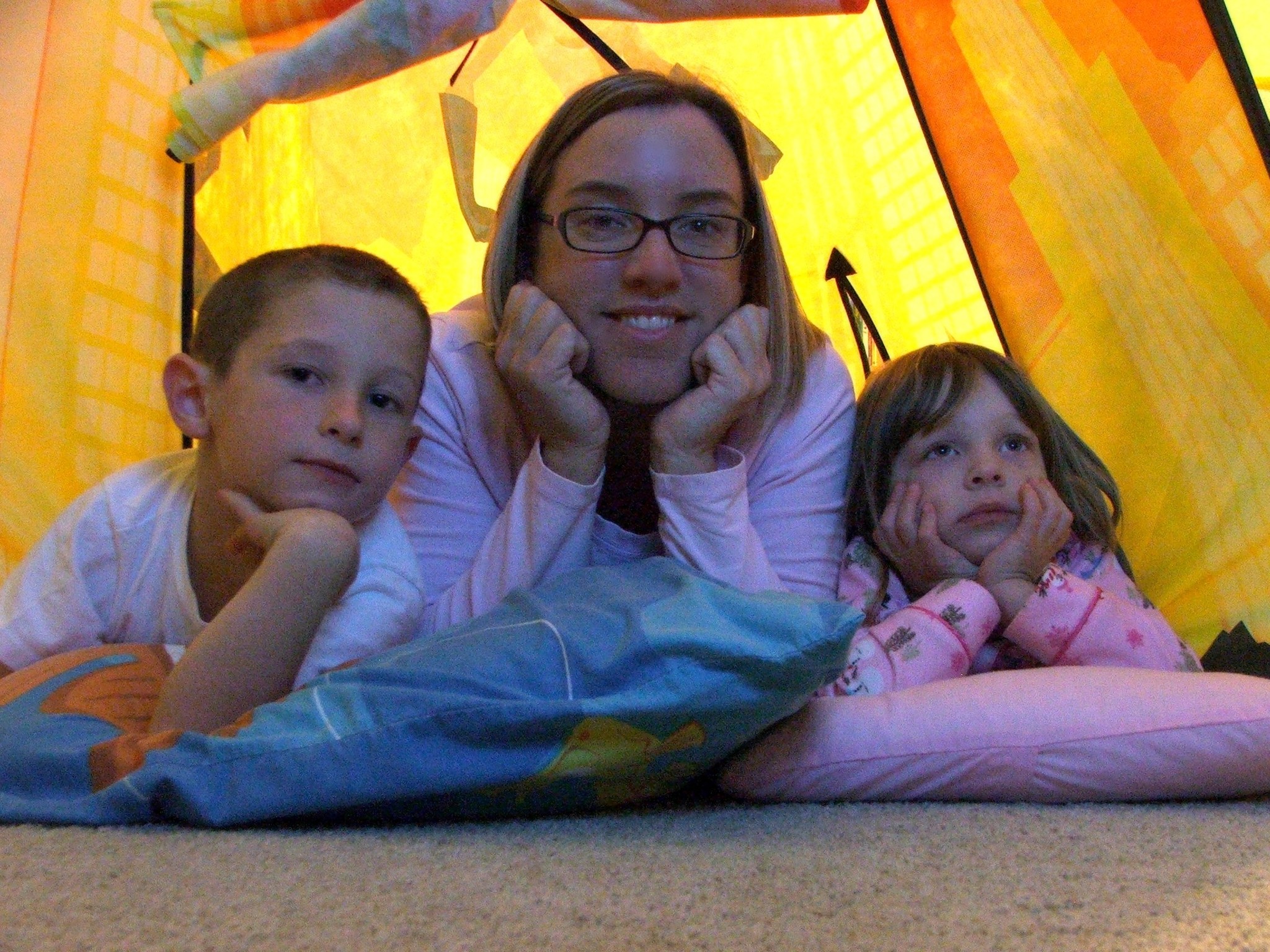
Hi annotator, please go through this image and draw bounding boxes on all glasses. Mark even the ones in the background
[533,205,756,261]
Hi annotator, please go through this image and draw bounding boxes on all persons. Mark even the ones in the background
[815,343,1203,698]
[387,68,857,640]
[0,244,433,736]
